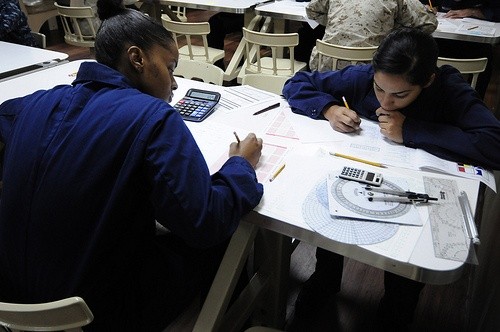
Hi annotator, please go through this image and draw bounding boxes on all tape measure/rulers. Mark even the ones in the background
[422,174,481,268]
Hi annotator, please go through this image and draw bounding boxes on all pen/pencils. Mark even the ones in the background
[233,131,241,144]
[253,102,281,116]
[342,96,350,111]
[268,163,285,182]
[429,0,433,9]
[467,25,479,30]
[329,151,388,169]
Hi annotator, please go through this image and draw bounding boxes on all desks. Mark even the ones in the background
[0,40,69,77]
[0,62,482,331]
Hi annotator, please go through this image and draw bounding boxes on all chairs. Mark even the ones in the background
[438,57,488,90]
[241,29,307,96]
[160,14,225,65]
[316,40,379,72]
[54,2,97,48]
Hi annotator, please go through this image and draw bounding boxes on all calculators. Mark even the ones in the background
[172,88,222,123]
[339,165,383,187]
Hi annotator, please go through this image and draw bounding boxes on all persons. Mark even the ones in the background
[61,0,128,40]
[305,0,438,73]
[420,0,500,99]
[0,0,264,332]
[283,28,500,332]
[0,0,35,48]
[206,10,265,72]
